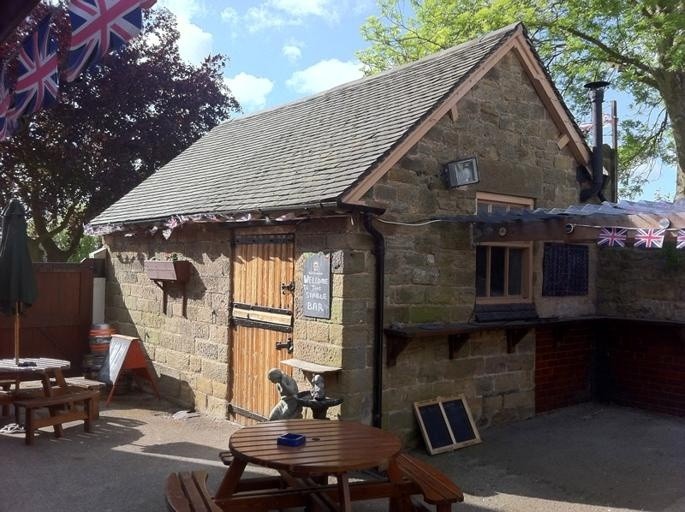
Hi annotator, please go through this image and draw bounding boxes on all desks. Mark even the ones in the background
[215,419,403,511]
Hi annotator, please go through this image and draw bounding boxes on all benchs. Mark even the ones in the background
[165,451,465,511]
[0,356,104,445]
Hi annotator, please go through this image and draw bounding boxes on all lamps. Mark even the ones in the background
[445,152,480,191]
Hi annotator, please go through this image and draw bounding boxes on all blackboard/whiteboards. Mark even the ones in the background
[302,250,331,320]
[413,396,483,451]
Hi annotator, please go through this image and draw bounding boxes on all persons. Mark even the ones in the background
[268,369,298,420]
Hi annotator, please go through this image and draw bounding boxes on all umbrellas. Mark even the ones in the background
[0,199,37,425]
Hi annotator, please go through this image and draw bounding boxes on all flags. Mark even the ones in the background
[0,66,14,138]
[632,228,665,249]
[7,18,59,119]
[677,229,685,249]
[597,227,628,248]
[63,1,141,83]
